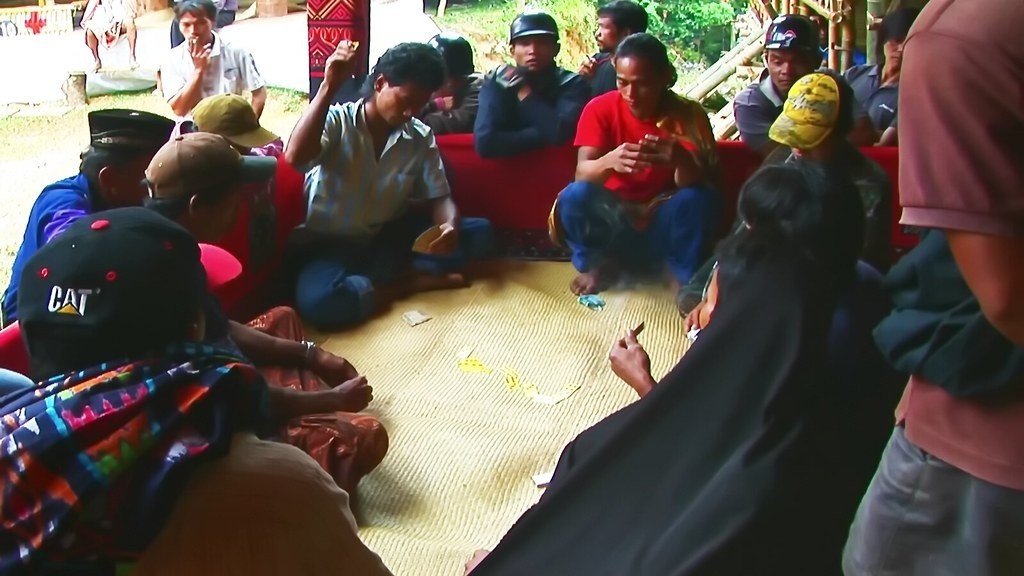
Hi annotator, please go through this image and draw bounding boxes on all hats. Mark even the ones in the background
[144,131,277,199]
[767,72,853,150]
[88,109,175,155]
[17,208,243,386]
[190,91,282,149]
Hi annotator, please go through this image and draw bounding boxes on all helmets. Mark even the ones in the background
[427,35,475,76]
[764,13,821,53]
[510,11,559,40]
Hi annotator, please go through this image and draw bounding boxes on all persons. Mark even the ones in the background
[0,0,1024,575]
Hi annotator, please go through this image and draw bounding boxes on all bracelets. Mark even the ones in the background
[299,340,316,367]
